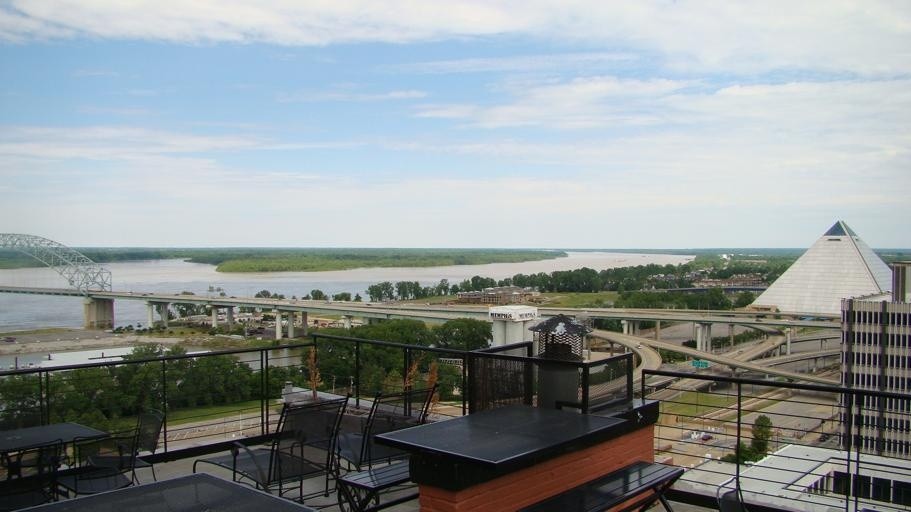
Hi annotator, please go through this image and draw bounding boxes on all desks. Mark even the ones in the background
[8,472,325,512]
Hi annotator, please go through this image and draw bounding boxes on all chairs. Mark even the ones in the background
[1,400,165,500]
[191,383,441,507]
